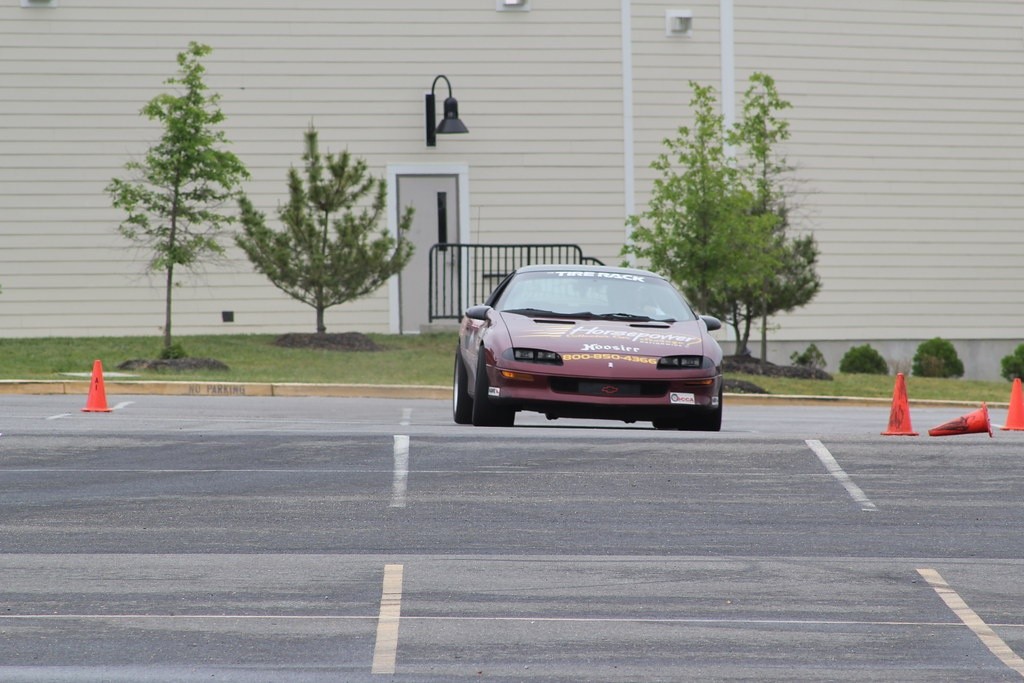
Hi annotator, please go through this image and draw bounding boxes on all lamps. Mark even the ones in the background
[425,75,470,147]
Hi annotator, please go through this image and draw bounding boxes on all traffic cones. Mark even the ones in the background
[880,372,920,436]
[928,400,993,438]
[999,377,1024,430]
[81,359,113,413]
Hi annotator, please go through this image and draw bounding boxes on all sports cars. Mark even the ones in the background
[452,263,725,432]
